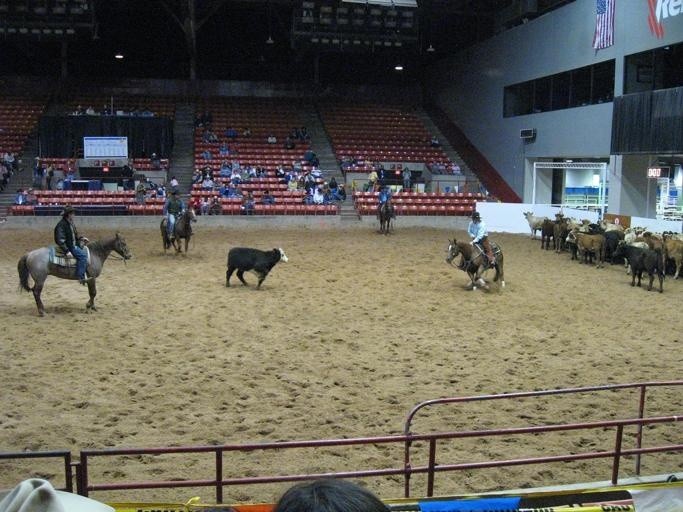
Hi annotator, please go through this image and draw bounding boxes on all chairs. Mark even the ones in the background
[3,84,490,218]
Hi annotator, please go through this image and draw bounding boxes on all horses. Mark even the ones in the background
[378,191,392,235]
[159,204,199,257]
[445,235,508,290]
[16,229,134,315]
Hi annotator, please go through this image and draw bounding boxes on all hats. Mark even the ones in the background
[0,479,115,512]
[171,189,184,195]
[469,213,482,217]
[382,185,389,189]
[60,205,75,216]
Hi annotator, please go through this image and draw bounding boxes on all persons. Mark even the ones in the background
[54,206,88,283]
[275,480,390,512]
[1,103,462,219]
[164,190,185,240]
[468,213,495,266]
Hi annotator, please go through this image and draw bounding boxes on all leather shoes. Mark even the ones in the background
[80,276,92,283]
[169,234,175,240]
[488,260,493,265]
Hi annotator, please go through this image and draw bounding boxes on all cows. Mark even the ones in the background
[223,245,289,290]
[522,209,682,293]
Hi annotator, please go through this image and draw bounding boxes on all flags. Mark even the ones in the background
[592,0,614,49]
